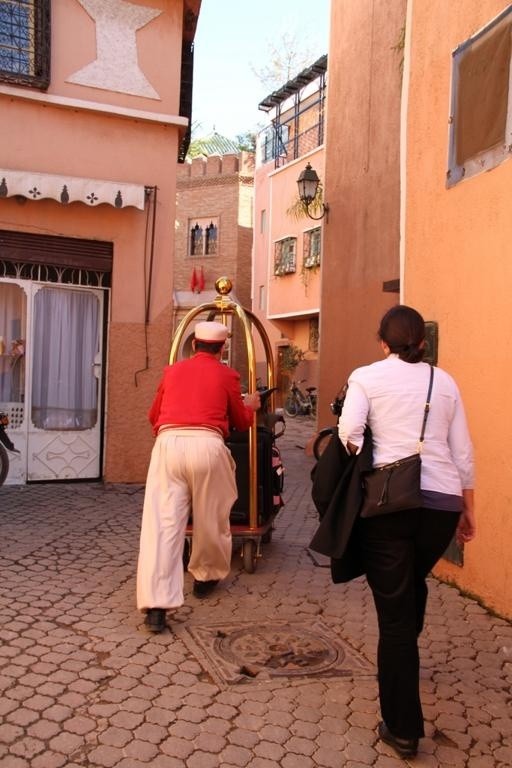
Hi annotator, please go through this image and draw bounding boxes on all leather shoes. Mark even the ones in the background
[143,609,167,632]
[192,579,219,598]
[379,722,419,758]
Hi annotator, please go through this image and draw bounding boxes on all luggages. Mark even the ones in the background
[226,426,286,524]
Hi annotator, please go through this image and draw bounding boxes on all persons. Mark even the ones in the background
[135,319,262,633]
[336,303,478,760]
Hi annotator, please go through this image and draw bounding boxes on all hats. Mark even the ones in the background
[195,322,228,345]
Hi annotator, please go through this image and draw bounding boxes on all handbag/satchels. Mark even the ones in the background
[360,453,427,518]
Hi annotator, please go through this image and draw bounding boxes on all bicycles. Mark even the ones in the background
[282,378,317,418]
[313,386,347,460]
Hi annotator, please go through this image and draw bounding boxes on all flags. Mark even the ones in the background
[199,269,206,291]
[190,269,198,293]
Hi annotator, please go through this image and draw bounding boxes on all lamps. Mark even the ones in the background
[296,160,331,227]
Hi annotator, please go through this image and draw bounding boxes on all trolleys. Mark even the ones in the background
[168,276,278,572]
